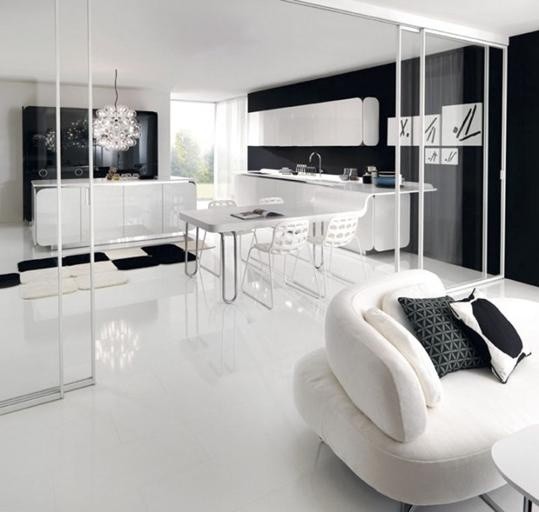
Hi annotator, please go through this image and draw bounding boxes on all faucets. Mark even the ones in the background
[309,151,321,174]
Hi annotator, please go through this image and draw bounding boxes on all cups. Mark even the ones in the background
[121,173,139,181]
[296,164,317,176]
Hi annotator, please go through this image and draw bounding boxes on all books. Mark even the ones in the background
[229,207,287,221]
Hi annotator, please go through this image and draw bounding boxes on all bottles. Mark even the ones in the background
[342,167,350,181]
[106,174,119,180]
[363,172,372,184]
[348,168,359,180]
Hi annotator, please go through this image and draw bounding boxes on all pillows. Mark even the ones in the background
[364,282,532,408]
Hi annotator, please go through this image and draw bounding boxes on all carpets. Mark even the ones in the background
[0,238,216,300]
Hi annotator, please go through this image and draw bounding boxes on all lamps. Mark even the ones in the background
[92,70,141,152]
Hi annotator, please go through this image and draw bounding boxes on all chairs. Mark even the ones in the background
[295,269,539,512]
[197,196,358,309]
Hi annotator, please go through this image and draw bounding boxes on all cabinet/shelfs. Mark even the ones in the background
[35,183,196,246]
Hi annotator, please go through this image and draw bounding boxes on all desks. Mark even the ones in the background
[179,201,358,304]
